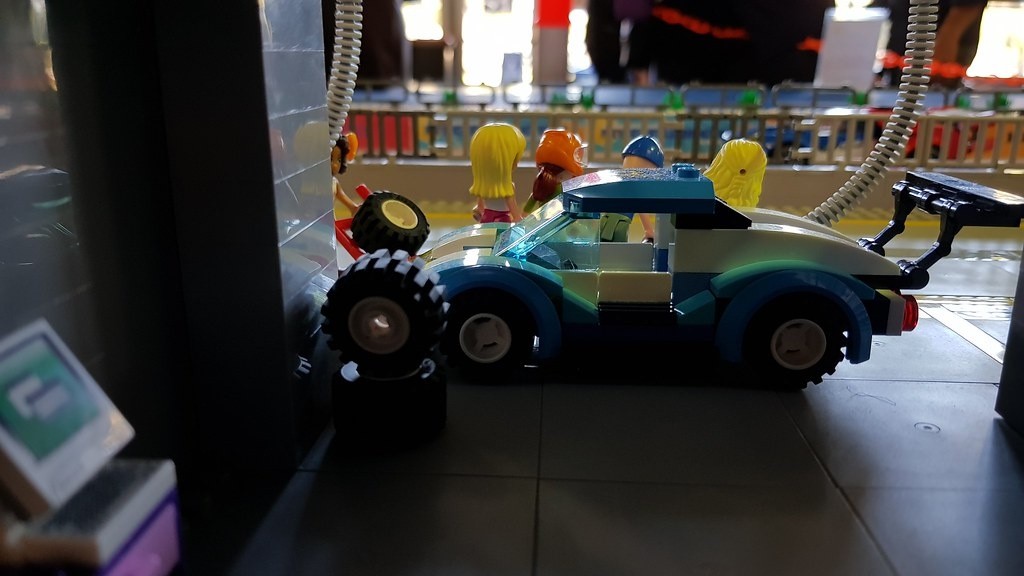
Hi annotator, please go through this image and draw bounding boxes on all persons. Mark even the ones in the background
[522,129,589,238]
[331,133,359,216]
[867,0,989,91]
[585,0,836,86]
[596,135,665,242]
[318,0,411,88]
[468,122,526,222]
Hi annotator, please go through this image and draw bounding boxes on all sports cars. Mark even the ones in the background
[321,160,1024,400]
